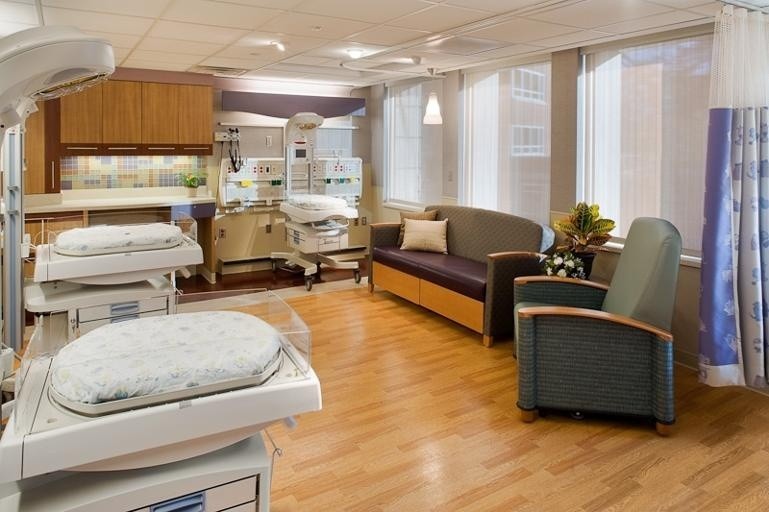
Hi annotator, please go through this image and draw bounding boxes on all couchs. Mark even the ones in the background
[367,203,542,348]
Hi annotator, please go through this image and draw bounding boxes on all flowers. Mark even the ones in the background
[544,252,587,282]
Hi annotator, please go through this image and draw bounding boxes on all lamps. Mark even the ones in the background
[422,74,443,125]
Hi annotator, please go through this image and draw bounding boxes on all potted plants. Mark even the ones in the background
[552,201,616,278]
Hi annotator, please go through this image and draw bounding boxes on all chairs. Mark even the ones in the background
[514,217,683,437]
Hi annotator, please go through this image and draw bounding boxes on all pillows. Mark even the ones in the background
[396,208,450,252]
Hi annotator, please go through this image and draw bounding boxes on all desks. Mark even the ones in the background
[21,186,217,284]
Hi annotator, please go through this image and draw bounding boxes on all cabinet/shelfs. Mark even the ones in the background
[141,81,214,145]
[59,78,141,144]
